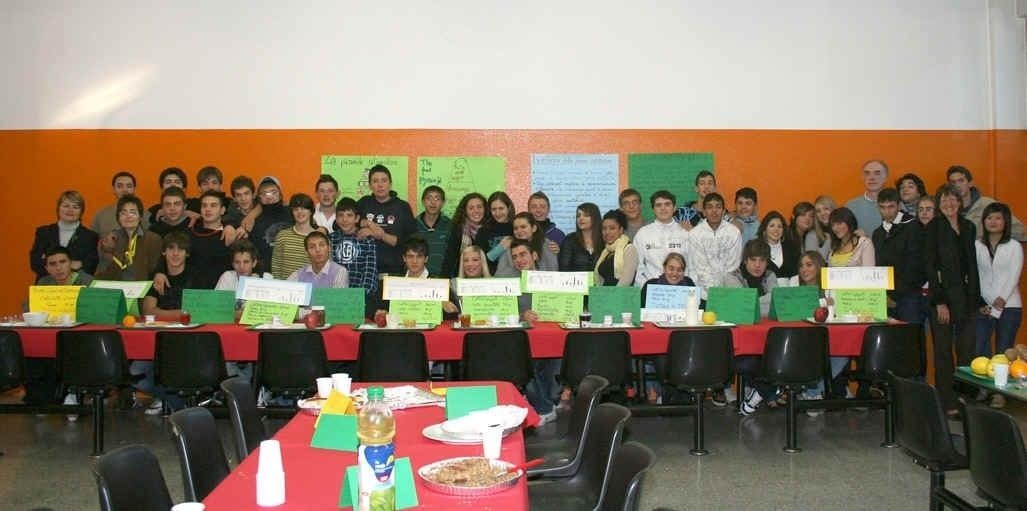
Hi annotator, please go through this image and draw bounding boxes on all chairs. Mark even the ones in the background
[594,441,652,511]
[56,329,146,459]
[461,326,530,396]
[171,406,233,501]
[886,368,971,510]
[154,330,237,411]
[0,330,46,394]
[253,330,329,415]
[528,401,631,508]
[762,325,830,453]
[855,324,926,446]
[89,446,174,511]
[524,371,610,482]
[963,404,1027,510]
[660,325,735,456]
[556,333,633,407]
[352,329,430,382]
[219,380,268,460]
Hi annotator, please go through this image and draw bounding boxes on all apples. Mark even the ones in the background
[179,312,191,325]
[987,363,995,377]
[303,313,320,329]
[375,313,387,327]
[991,354,1011,375]
[971,356,991,375]
[814,307,829,323]
[702,311,716,325]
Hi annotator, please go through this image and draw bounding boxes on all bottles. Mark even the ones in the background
[356,385,396,511]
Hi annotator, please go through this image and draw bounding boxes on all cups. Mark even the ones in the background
[457,315,471,330]
[305,311,321,329]
[703,312,717,326]
[331,379,350,396]
[145,315,155,326]
[180,311,190,328]
[57,315,72,328]
[318,377,332,400]
[254,439,286,506]
[578,314,591,329]
[483,428,502,461]
[604,316,613,327]
[506,314,521,326]
[172,502,206,511]
[815,309,828,323]
[620,313,633,326]
[994,364,1009,387]
[375,311,389,328]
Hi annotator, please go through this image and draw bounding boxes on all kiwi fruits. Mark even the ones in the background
[1016,344,1025,354]
[1004,348,1018,361]
[1021,346,1027,361]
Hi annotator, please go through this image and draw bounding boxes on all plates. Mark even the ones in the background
[418,456,522,499]
[422,422,519,445]
[297,397,332,416]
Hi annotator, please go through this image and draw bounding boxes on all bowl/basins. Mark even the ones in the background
[23,313,49,326]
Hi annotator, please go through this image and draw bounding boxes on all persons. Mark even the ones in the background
[331,198,375,293]
[313,176,342,230]
[450,193,496,255]
[32,249,95,418]
[635,191,693,295]
[212,244,269,411]
[156,168,185,199]
[924,188,979,422]
[271,192,327,278]
[97,174,150,237]
[691,194,744,406]
[804,197,839,264]
[352,164,415,321]
[414,186,464,276]
[194,165,263,234]
[899,173,934,223]
[187,192,239,288]
[596,211,638,289]
[557,204,605,272]
[790,252,835,418]
[472,192,518,274]
[244,177,294,275]
[641,253,707,406]
[146,188,194,243]
[133,235,200,415]
[673,172,719,227]
[527,194,566,270]
[618,189,645,242]
[843,161,889,234]
[229,175,257,215]
[510,241,571,421]
[757,211,799,287]
[825,208,877,404]
[870,190,920,403]
[976,203,1020,410]
[287,233,349,411]
[496,210,557,276]
[36,191,97,272]
[917,193,937,224]
[95,197,161,281]
[377,240,460,384]
[949,166,1020,244]
[730,186,760,253]
[726,241,788,416]
[789,201,813,252]
[446,244,514,409]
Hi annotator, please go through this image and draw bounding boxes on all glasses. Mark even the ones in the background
[118,210,138,216]
[423,195,443,202]
[258,190,281,197]
[917,206,937,213]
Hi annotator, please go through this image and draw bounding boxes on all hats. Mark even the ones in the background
[257,175,281,190]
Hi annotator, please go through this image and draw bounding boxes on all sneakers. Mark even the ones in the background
[802,391,826,418]
[62,394,81,422]
[711,389,727,409]
[869,382,885,399]
[724,389,737,407]
[739,388,763,418]
[144,396,165,416]
[538,406,557,427]
